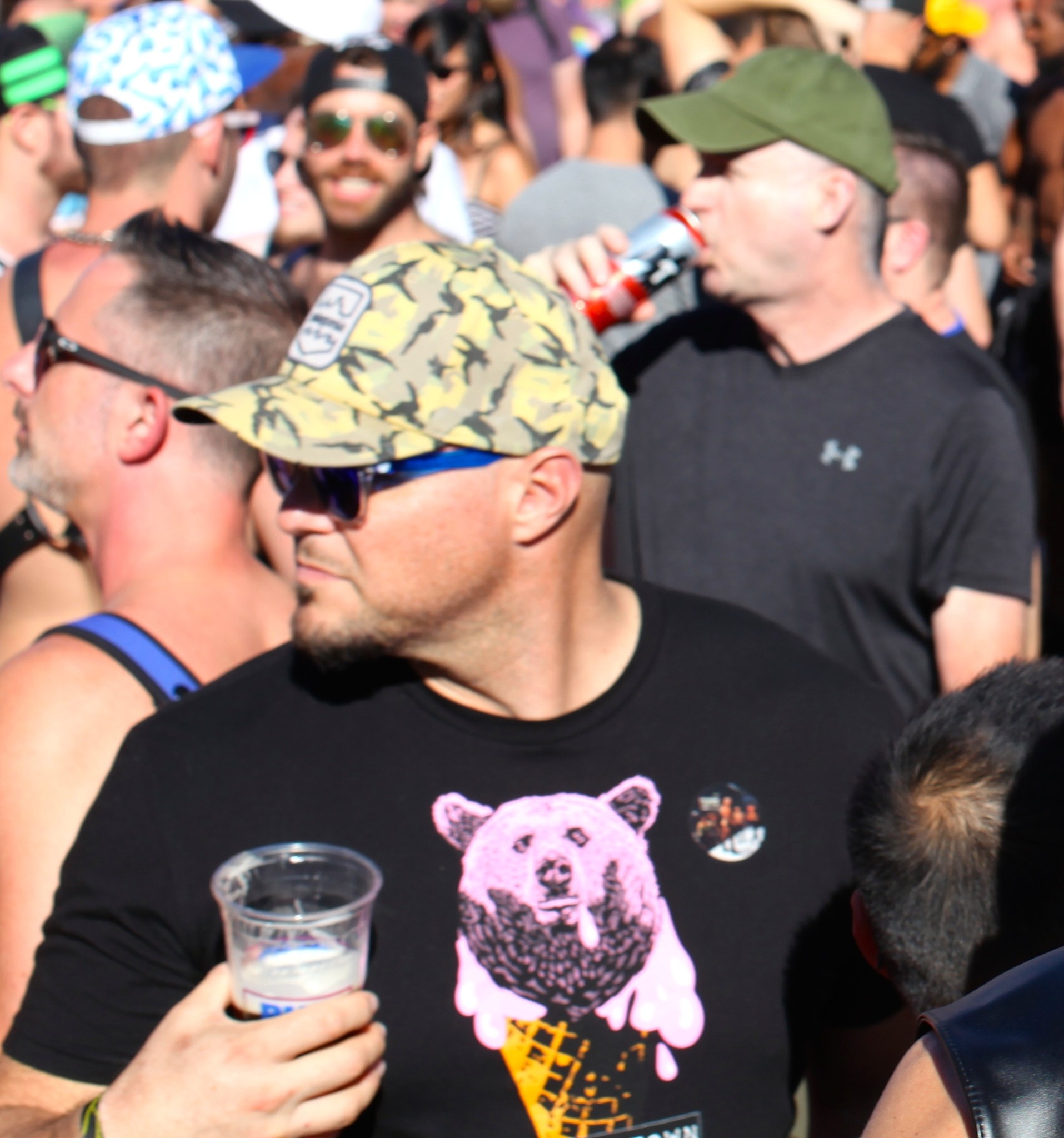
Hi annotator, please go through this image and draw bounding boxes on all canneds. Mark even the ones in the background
[557,209,708,336]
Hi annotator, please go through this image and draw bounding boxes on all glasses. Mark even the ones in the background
[304,109,415,156]
[33,320,194,400]
[265,448,509,527]
[429,66,475,79]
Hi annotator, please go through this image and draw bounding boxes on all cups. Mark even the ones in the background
[210,843,383,1022]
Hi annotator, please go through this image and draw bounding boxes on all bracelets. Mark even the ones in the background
[80,1094,100,1138]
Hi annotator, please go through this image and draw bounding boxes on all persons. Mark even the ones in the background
[2,0,1062,1137]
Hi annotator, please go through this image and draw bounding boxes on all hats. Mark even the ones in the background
[65,1,282,145]
[633,44,901,195]
[168,239,631,468]
[860,64,982,174]
[925,0,991,38]
[302,36,426,126]
